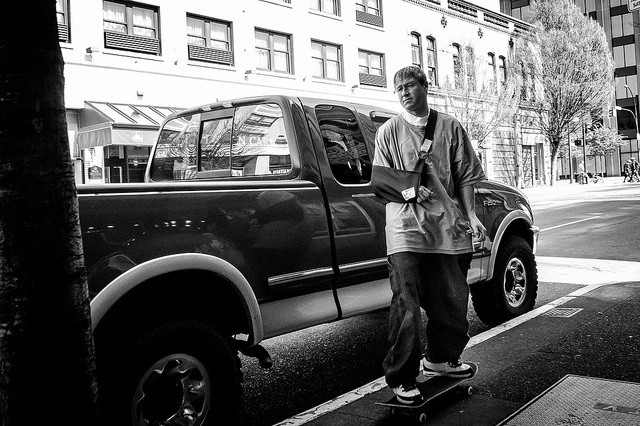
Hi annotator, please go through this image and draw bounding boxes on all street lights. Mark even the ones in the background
[614,84,640,164]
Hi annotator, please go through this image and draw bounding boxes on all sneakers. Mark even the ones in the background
[422,356,474,378]
[393,383,424,405]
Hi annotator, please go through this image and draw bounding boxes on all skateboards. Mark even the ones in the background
[374,361,479,423]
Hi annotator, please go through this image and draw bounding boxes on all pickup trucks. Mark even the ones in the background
[77,95,539,426]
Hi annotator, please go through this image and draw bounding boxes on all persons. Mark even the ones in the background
[627,157,640,182]
[578,160,599,184]
[622,159,635,182]
[369,63,489,404]
